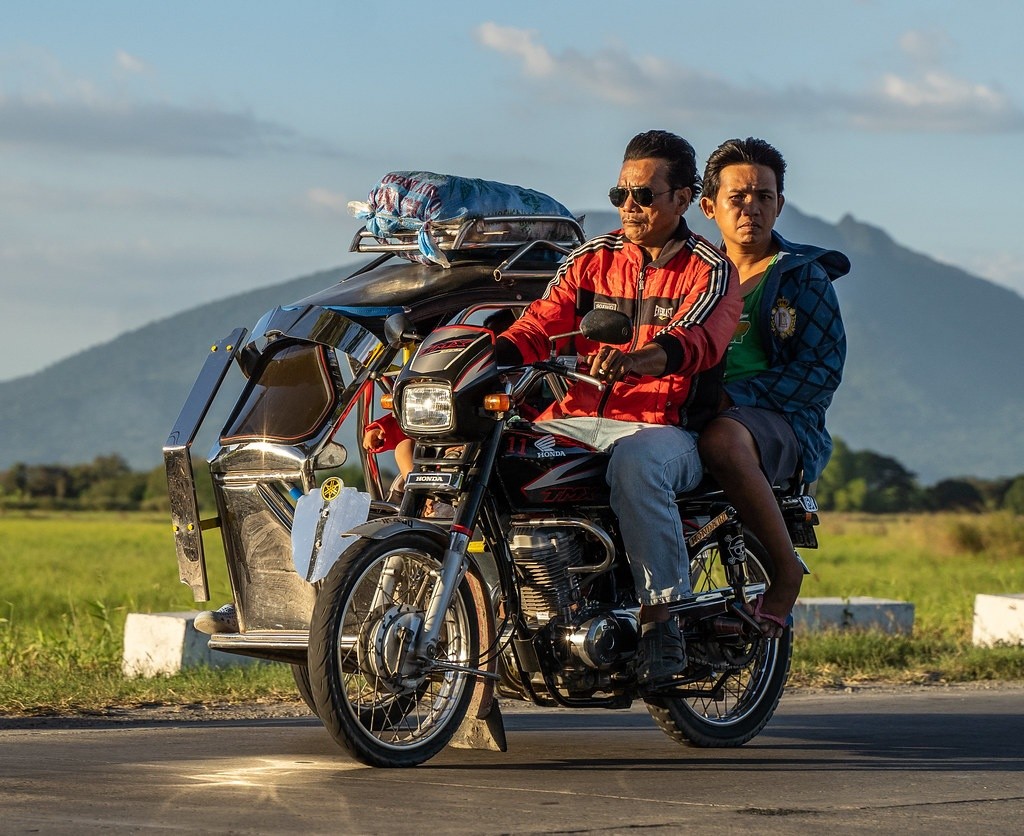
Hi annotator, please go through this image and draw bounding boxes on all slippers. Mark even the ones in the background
[730,593,787,636]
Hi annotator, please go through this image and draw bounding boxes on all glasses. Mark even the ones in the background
[608,186,681,207]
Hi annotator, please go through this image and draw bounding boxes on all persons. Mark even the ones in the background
[365,417,416,481]
[693,134,850,628]
[495,131,741,672]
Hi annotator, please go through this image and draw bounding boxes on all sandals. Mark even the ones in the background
[636,612,687,683]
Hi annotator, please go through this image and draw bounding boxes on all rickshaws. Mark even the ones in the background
[163,209,819,769]
